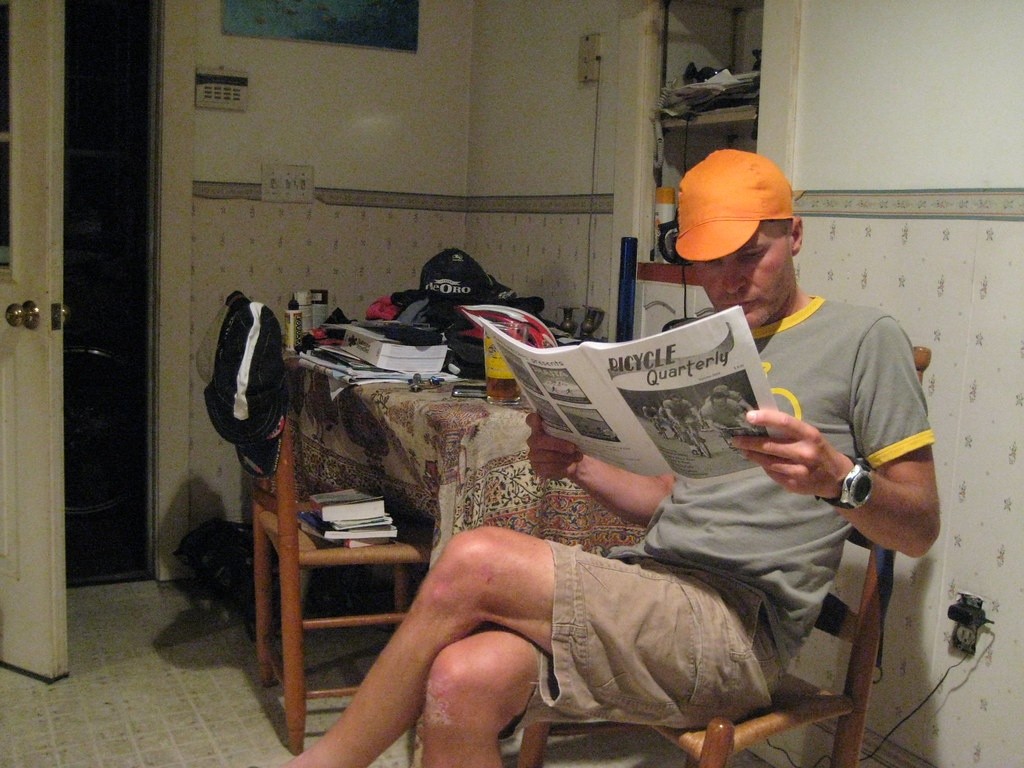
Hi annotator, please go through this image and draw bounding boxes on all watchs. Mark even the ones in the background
[814,454,874,509]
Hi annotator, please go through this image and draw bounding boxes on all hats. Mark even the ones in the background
[674,148,794,261]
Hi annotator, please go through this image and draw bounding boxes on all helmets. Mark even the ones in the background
[444,304,559,363]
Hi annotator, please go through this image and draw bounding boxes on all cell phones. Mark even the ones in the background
[452,385,487,399]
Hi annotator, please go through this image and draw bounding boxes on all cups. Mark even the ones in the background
[483,321,528,406]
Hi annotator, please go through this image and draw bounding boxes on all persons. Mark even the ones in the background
[658,393,706,445]
[701,385,754,427]
[642,402,667,434]
[279,149,941,768]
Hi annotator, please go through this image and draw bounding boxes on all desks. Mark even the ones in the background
[267,353,651,580]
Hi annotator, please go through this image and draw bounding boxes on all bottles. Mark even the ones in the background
[654,187,676,264]
[285,290,303,351]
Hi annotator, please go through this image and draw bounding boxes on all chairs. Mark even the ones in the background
[249,359,432,755]
[518,347,934,768]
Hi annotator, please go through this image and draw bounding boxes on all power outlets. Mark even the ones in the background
[260,163,315,203]
[577,34,601,82]
[949,590,989,657]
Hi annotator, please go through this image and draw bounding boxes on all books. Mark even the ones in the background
[297,488,397,549]
[478,305,785,479]
[340,330,448,372]
